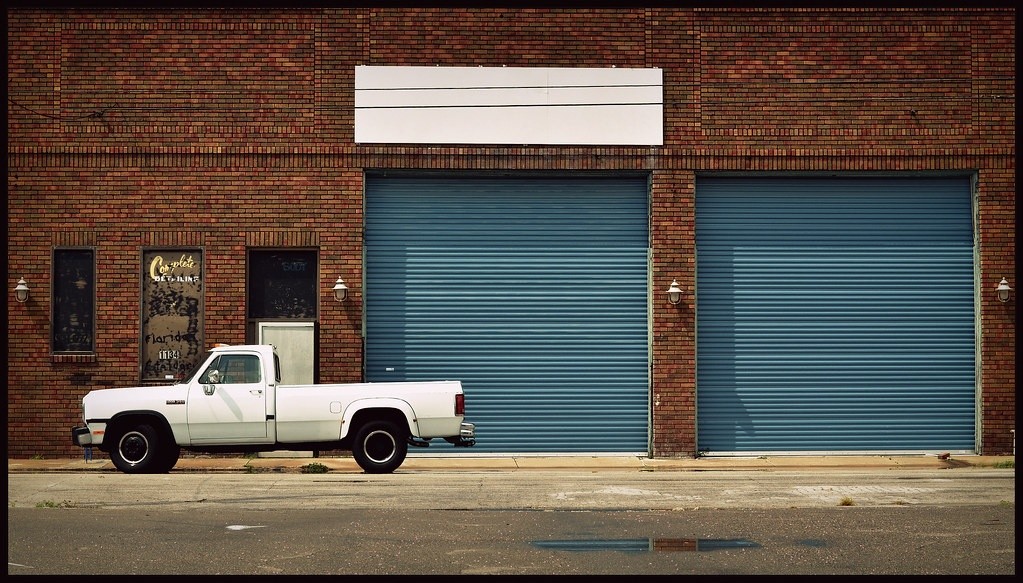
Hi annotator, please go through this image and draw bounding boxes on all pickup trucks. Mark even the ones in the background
[69,342,476,476]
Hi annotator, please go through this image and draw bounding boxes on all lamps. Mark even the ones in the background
[666,278,683,306]
[332,276,348,303]
[995,276,1014,304]
[13,277,30,302]
